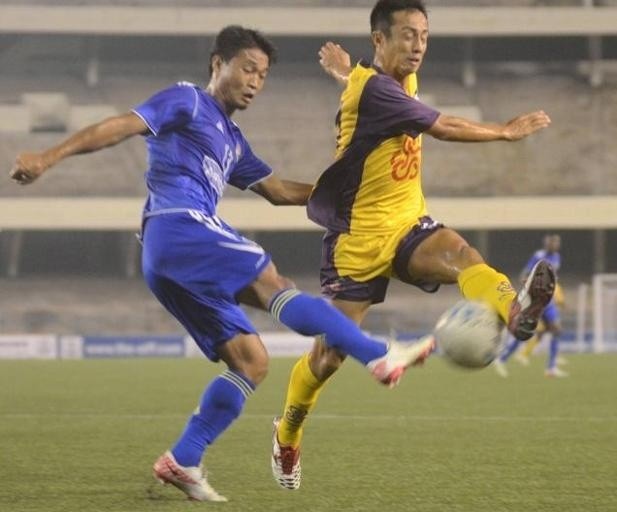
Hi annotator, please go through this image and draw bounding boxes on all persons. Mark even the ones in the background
[493,235,569,378]
[10,25,436,502]
[514,284,564,364]
[271,1,556,491]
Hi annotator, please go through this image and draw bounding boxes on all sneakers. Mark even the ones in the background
[149,448,229,505]
[490,352,570,377]
[364,332,438,390]
[505,256,557,342]
[270,416,306,492]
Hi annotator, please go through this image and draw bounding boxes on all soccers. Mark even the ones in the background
[436,301,506,368]
